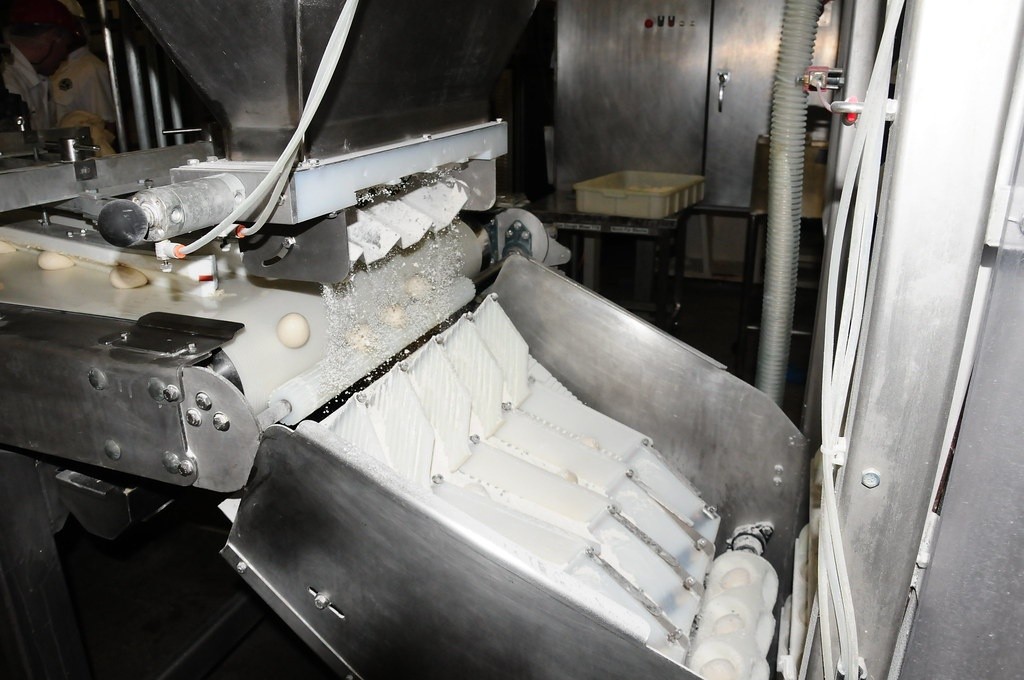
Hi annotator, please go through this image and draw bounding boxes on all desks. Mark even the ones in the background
[523,191,690,328]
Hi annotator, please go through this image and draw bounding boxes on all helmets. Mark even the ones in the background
[8,0,87,46]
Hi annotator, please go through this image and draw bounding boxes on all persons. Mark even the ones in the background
[0,0,117,146]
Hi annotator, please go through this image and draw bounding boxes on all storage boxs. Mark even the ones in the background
[573,170,706,219]
